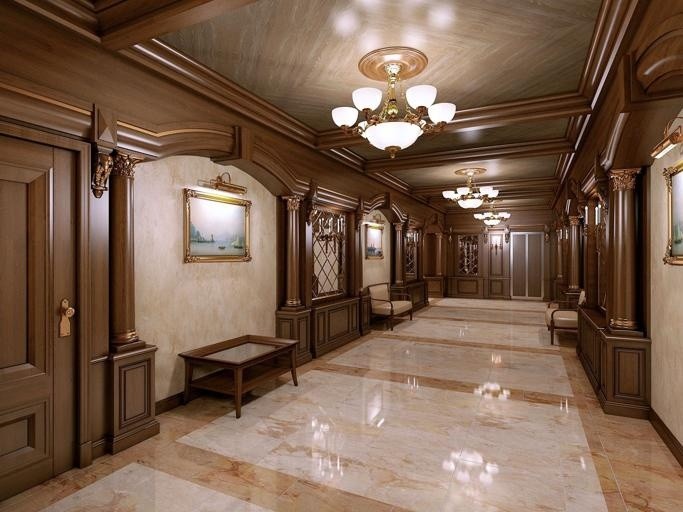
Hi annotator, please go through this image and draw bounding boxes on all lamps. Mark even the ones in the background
[442,168,499,209]
[474,204,511,228]
[331,45,456,161]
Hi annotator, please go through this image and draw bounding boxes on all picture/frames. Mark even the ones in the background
[365,224,384,260]
[183,189,252,262]
[662,160,683,266]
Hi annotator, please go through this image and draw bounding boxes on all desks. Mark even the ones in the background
[178,335,300,419]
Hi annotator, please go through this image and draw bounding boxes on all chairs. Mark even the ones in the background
[368,282,412,330]
[546,290,586,345]
